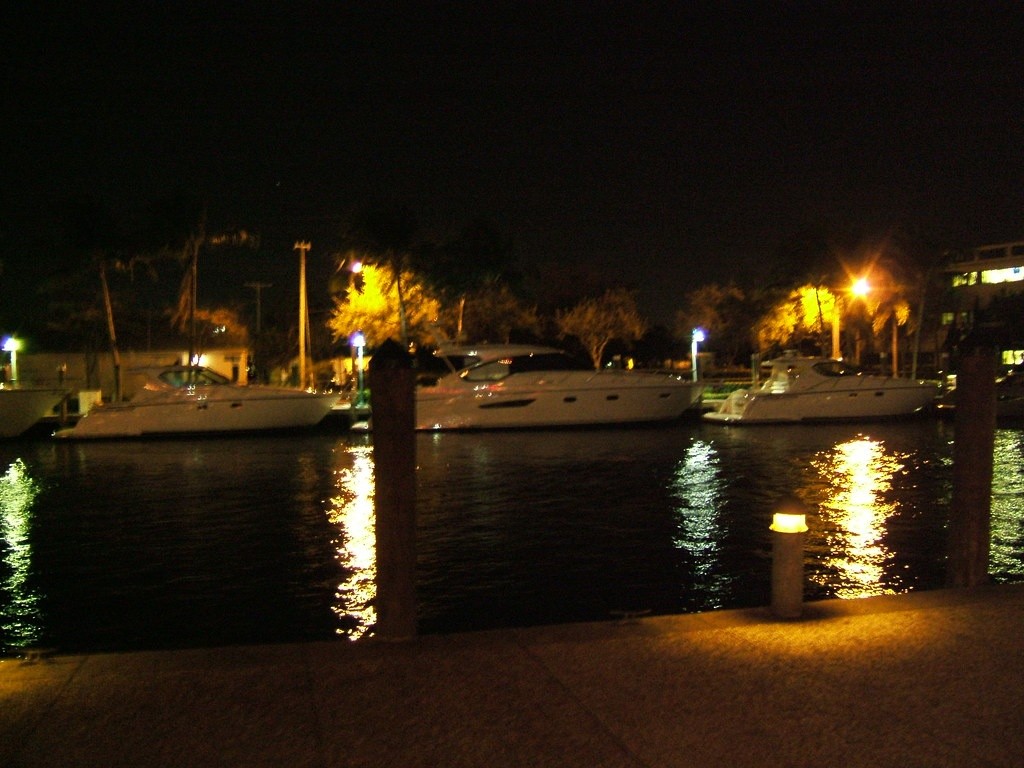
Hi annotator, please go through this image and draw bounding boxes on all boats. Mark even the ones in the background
[0,387,74,440]
[50,366,345,438]
[701,357,939,423]
[415,344,692,432]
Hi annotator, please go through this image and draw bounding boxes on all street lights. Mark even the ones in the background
[352,333,367,392]
[690,328,704,382]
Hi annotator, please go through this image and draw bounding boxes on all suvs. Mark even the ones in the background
[942,241,1022,274]
